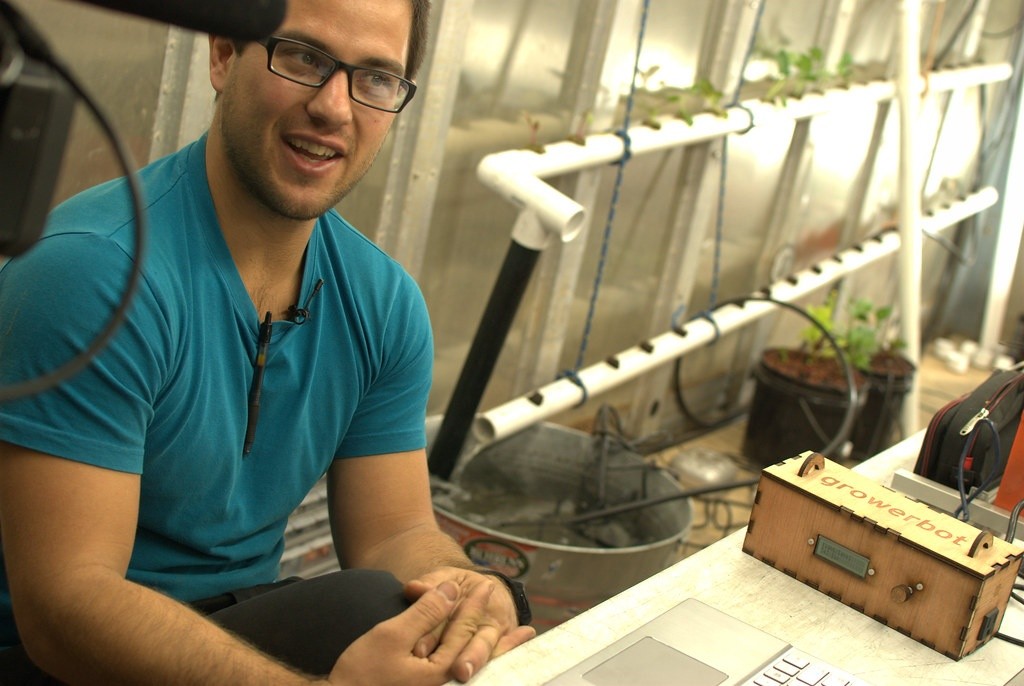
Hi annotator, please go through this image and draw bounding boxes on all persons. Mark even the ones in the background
[0,0,538,686]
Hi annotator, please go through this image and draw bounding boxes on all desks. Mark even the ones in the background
[418,420,1024,686]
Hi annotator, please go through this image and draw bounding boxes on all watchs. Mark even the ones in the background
[473,568,531,628]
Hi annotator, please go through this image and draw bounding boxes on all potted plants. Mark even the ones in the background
[737,287,876,473]
[837,296,918,463]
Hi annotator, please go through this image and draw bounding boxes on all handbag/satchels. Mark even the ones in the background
[915,368,1024,493]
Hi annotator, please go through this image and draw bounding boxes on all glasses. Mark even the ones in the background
[259,35,417,114]
